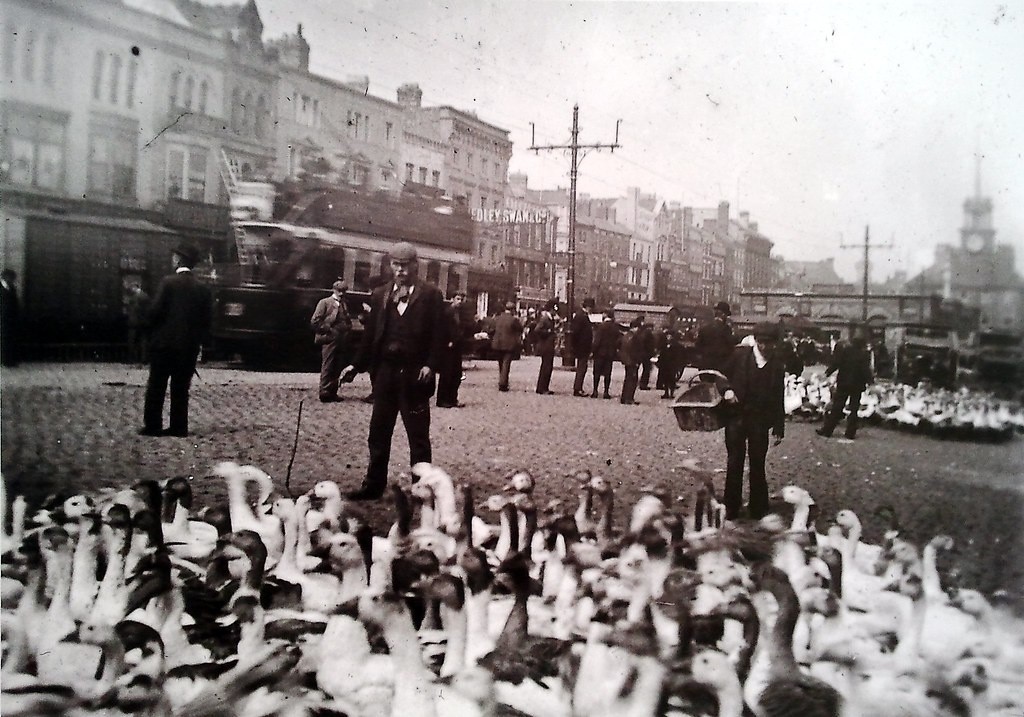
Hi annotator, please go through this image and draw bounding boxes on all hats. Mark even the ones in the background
[716,302,732,316]
[390,241,416,262]
[605,307,615,317]
[333,281,348,291]
[752,322,779,341]
[584,297,595,306]
[170,240,202,262]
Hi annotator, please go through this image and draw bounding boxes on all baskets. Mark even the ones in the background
[667,369,728,431]
[479,314,495,352]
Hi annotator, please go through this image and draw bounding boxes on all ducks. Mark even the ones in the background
[0,470,1023,716]
[783,373,1024,441]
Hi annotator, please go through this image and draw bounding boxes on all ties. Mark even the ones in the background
[333,298,351,324]
[398,286,409,303]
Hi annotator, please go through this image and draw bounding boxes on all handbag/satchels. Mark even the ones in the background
[533,332,556,356]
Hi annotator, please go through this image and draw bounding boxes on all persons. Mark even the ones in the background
[345,241,445,501]
[437,291,466,409]
[310,280,351,402]
[714,313,787,520]
[815,322,874,441]
[0,269,22,368]
[473,297,834,405]
[135,243,212,438]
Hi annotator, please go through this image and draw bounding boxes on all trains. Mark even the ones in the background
[193,172,474,372]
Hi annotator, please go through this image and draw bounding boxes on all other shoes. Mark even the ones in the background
[138,427,164,436]
[536,388,553,394]
[320,393,346,401]
[164,428,188,437]
[620,398,641,405]
[347,486,383,500]
[845,434,855,438]
[574,389,588,397]
[817,429,830,437]
[437,401,453,407]
[360,394,375,402]
[449,400,466,407]
